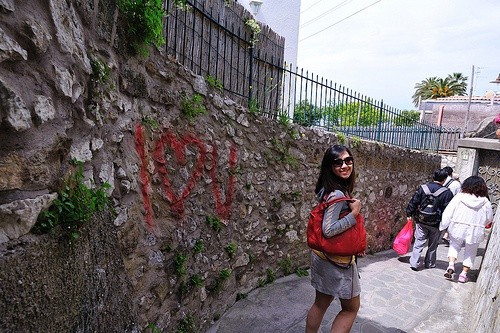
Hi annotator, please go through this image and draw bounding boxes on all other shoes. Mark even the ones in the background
[429,262,435,268]
[442,232,449,246]
[410,264,420,271]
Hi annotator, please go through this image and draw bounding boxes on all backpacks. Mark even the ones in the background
[414,184,449,226]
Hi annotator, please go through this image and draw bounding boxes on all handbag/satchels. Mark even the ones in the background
[392,220,413,255]
[306,188,367,256]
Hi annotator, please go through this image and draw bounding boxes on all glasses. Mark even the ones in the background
[330,156,353,165]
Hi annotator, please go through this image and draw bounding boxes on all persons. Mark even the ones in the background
[306,145,366,333]
[441,167,463,241]
[406,170,453,270]
[439,176,494,283]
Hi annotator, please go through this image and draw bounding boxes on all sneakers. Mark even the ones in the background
[458,271,468,283]
[444,265,455,279]
